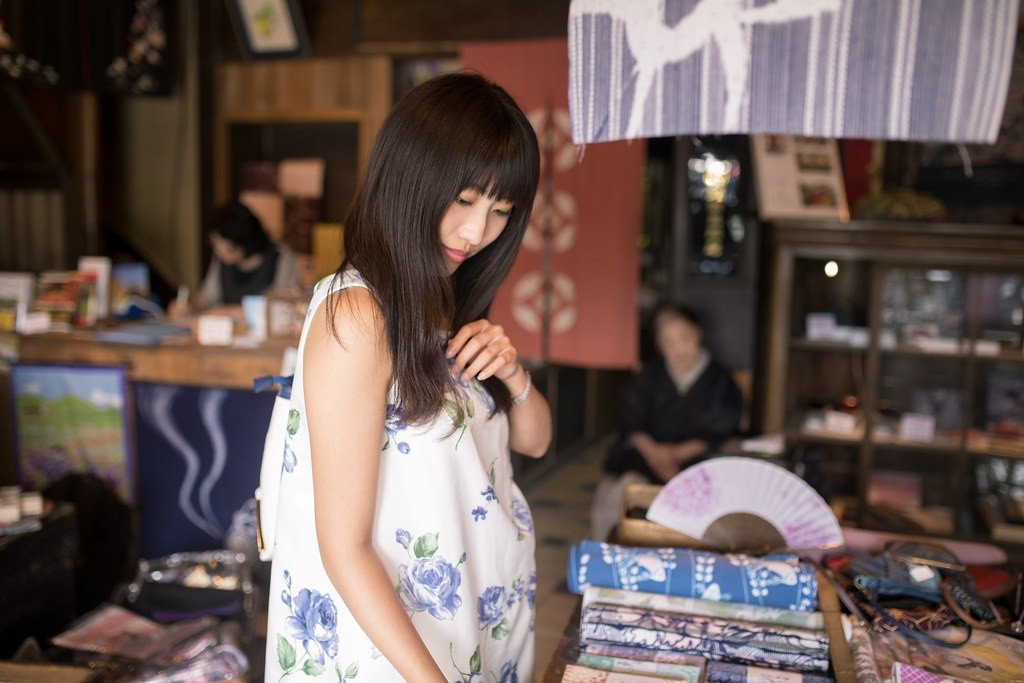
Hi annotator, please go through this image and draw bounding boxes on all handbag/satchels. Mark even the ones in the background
[845,540,1004,648]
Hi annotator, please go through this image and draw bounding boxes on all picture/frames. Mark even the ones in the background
[224,0,310,63]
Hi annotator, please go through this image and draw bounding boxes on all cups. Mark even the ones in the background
[806,313,837,339]
[899,416,936,440]
[77,257,111,319]
[242,296,269,341]
[824,411,856,433]
[197,313,234,346]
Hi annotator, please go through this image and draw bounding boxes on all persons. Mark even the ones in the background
[170,202,299,320]
[264,71,552,683]
[592,305,742,543]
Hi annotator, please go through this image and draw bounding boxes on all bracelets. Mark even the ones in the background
[510,369,532,406]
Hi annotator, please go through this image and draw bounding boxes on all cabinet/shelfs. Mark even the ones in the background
[758,219,1024,564]
